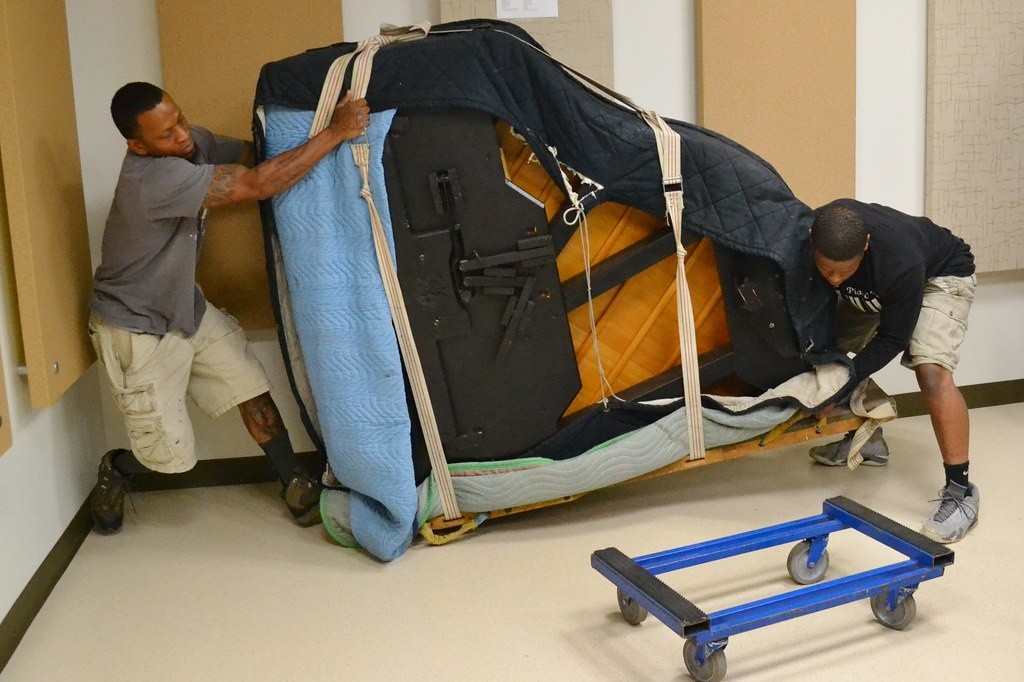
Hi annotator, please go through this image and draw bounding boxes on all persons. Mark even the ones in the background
[87,81,371,535]
[808,199,980,543]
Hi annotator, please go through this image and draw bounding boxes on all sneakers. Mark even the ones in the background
[922,481,979,544]
[90,449,138,533]
[280,470,323,525]
[809,427,889,468]
